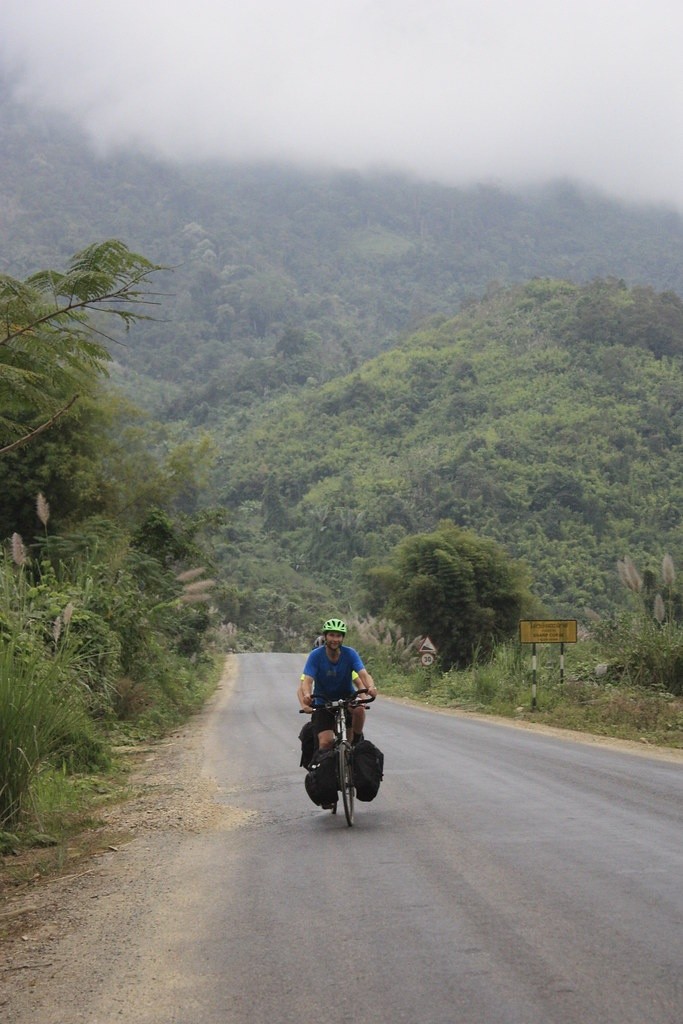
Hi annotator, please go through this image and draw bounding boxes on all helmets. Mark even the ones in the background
[315,636,326,648]
[323,619,347,633]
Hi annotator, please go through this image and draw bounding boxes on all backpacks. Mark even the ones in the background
[304,748,340,809]
[351,740,384,801]
[298,722,318,771]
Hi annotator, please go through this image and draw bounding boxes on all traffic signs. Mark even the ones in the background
[519,619,578,644]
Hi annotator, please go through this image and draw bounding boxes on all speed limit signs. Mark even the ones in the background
[421,652,435,666]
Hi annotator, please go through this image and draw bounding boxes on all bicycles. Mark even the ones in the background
[307,688,376,827]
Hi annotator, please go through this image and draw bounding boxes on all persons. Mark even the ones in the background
[297,617,377,811]
